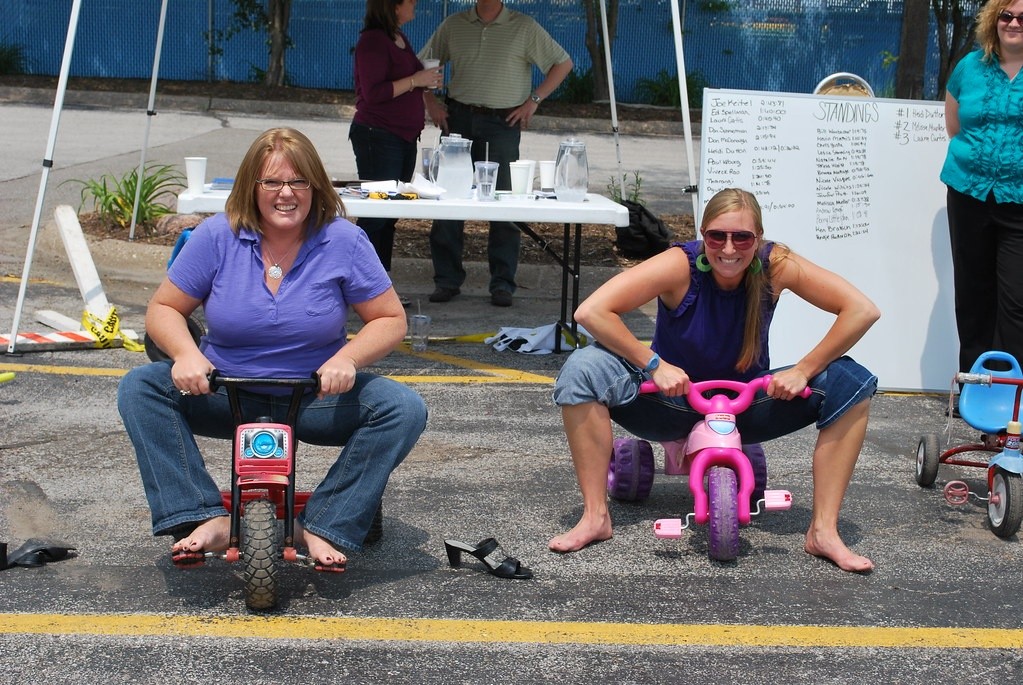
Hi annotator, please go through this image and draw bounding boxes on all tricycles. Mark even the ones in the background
[605,373,813,563]
[172,369,346,612]
[915,351,1023,538]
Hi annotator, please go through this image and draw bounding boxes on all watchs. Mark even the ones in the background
[531,93,541,105]
[644,353,659,372]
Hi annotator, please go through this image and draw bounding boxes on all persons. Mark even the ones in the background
[348,0,445,272]
[548,189,882,570]
[416,0,573,307]
[117,128,428,565]
[940,0,1023,417]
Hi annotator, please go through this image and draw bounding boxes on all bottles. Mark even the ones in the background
[1004,421,1021,458]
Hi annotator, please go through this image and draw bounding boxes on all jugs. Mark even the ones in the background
[429,133,473,196]
[554,138,588,202]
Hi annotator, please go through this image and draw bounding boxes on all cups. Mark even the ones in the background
[184,157,208,191]
[517,160,536,192]
[540,161,557,190]
[410,315,431,352]
[422,147,439,182]
[510,162,530,194]
[474,161,500,202]
[423,58,441,89]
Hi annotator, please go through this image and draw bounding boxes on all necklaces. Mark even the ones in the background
[264,238,299,280]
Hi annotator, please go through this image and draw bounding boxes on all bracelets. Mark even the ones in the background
[409,76,414,92]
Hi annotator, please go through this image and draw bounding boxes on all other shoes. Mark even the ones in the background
[947,402,961,417]
[398,295,411,306]
[431,284,461,303]
[491,290,512,307]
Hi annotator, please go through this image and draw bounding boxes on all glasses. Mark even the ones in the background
[702,230,761,251]
[255,178,314,191]
[996,11,1023,24]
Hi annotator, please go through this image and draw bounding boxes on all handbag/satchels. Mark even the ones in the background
[484,322,595,355]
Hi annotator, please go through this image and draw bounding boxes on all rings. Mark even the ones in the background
[180,390,191,395]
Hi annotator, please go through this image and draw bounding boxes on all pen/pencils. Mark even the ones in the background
[546,196,557,199]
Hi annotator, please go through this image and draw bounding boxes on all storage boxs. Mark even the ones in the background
[360,180,397,191]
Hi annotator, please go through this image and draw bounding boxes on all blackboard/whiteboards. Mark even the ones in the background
[697,85,964,391]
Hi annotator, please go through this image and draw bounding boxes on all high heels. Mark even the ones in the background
[0,542,76,568]
[444,537,532,579]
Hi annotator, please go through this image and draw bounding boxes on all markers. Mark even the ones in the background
[494,192,541,201]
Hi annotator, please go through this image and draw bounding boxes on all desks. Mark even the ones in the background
[177,183,630,356]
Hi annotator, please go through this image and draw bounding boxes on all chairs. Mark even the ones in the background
[959,350,1023,438]
